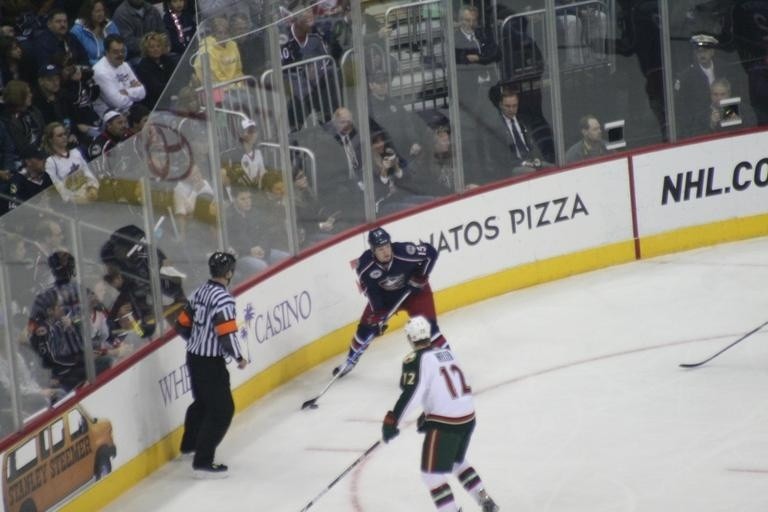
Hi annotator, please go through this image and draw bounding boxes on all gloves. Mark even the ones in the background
[382,411,400,443]
[367,312,387,337]
[406,274,429,294]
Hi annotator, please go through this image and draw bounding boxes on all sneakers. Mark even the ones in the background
[192,464,230,472]
[482,497,496,511]
[333,360,355,377]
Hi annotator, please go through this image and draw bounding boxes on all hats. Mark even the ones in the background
[36,63,63,76]
[368,70,388,83]
[238,119,256,132]
[20,144,49,158]
[690,34,718,48]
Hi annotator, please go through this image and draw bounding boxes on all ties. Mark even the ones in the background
[344,136,360,179]
[469,35,480,54]
[511,118,528,157]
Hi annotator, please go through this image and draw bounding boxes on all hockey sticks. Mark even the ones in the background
[301,289,412,409]
[679,322,768,367]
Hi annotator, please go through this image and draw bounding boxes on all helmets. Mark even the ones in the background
[209,252,236,278]
[404,315,432,343]
[368,227,390,247]
[48,250,75,275]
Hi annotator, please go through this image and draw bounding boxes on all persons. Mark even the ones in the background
[0,0,362,436]
[357,2,767,218]
[177,251,249,474]
[382,313,500,511]
[334,227,438,377]
